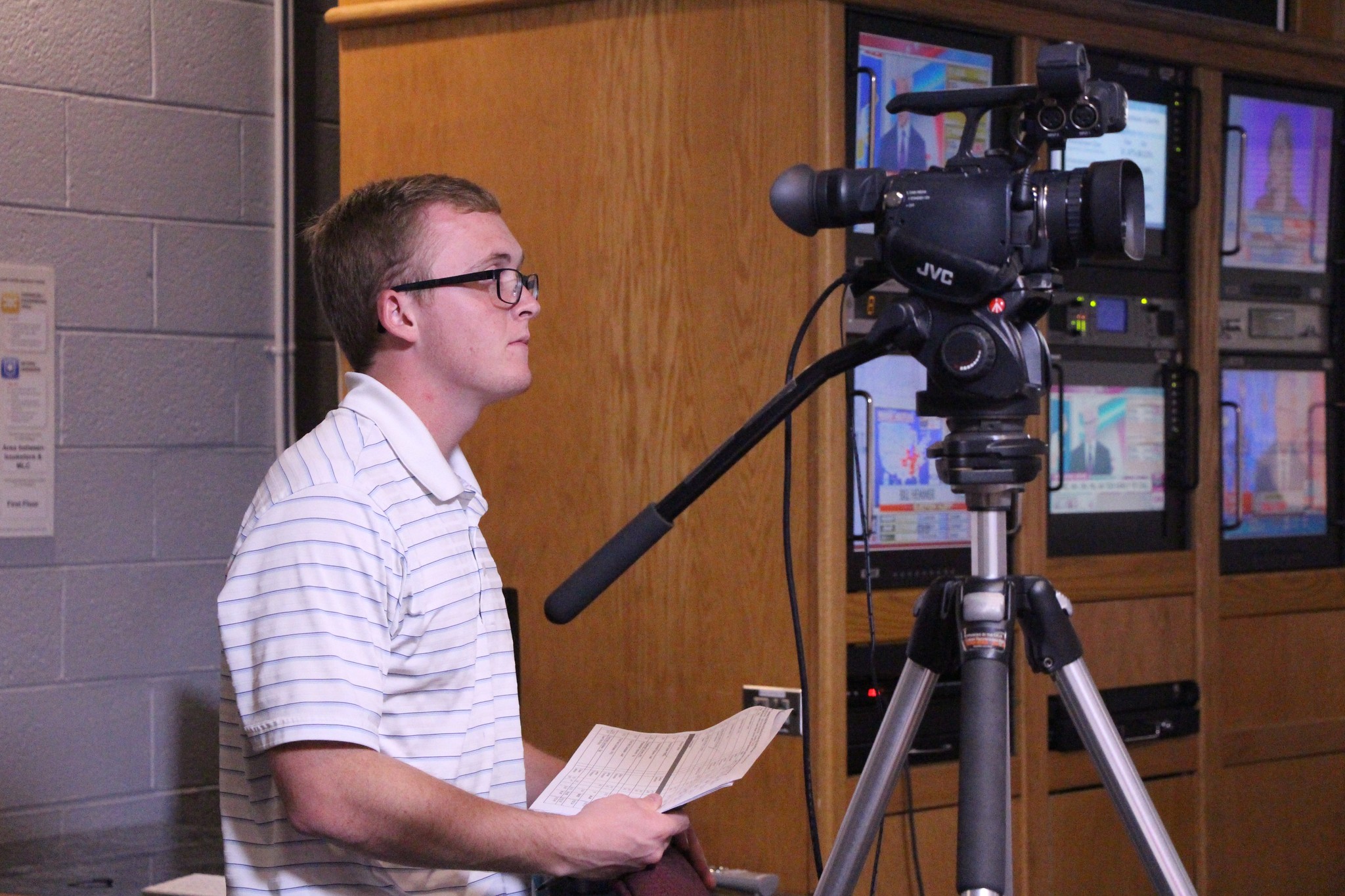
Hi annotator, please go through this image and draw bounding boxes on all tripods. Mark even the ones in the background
[794,295,1215,896]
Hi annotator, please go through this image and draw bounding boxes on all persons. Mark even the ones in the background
[878,70,927,171]
[1069,403,1113,475]
[213,174,690,895]
[1256,121,1308,218]
[1257,375,1307,491]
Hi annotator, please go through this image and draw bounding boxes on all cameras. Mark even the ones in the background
[770,38,1147,289]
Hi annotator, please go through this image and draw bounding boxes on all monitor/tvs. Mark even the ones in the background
[842,9,1343,592]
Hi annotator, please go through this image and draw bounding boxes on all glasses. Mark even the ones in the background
[377,268,540,335]
